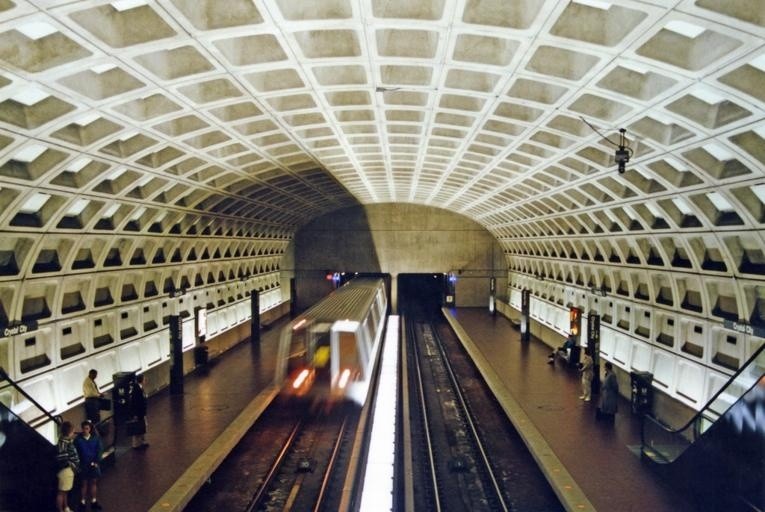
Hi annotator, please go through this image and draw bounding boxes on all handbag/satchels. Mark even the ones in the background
[99,397,112,412]
[39,443,67,473]
[125,419,146,437]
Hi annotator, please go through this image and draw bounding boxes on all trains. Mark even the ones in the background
[274,275,389,418]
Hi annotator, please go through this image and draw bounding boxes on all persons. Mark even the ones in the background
[56,420,80,512]
[547,334,573,367]
[601,361,618,428]
[132,375,150,448]
[576,349,594,401]
[74,422,102,511]
[82,369,105,422]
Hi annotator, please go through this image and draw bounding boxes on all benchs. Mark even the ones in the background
[557,345,583,375]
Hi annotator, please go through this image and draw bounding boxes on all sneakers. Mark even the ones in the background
[579,395,591,402]
[64,500,103,512]
